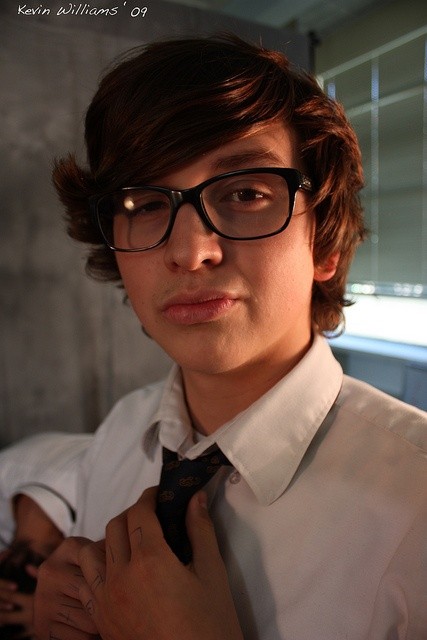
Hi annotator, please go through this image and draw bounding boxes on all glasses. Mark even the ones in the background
[89,166,313,252]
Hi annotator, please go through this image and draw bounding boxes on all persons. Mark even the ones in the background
[33,34,426,640]
[0,432,91,640]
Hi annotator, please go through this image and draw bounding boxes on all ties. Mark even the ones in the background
[151,442,231,566]
